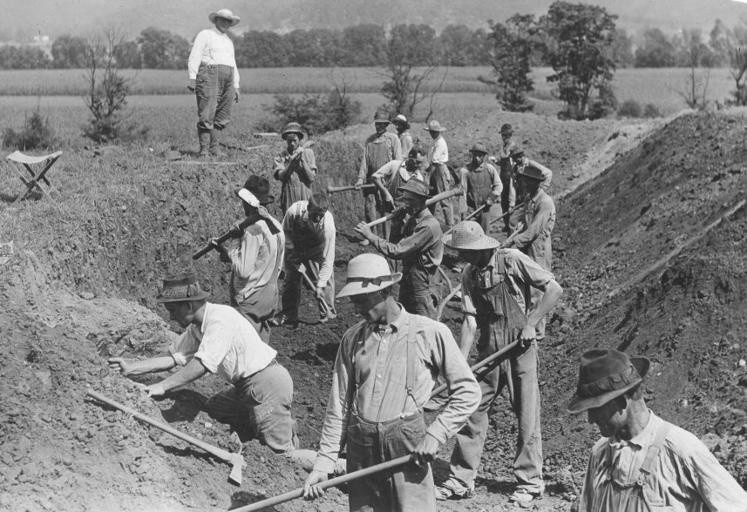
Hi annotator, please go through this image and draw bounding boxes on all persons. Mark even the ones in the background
[187,8,242,157]
[568,348,746,512]
[107,111,563,509]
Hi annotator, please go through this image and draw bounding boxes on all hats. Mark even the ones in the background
[396,176,432,199]
[440,219,501,251]
[498,123,515,136]
[468,142,488,157]
[566,348,650,414]
[155,274,210,302]
[423,119,448,133]
[392,114,411,129]
[334,252,403,300]
[372,111,391,124]
[508,145,524,157]
[208,8,241,27]
[234,174,275,205]
[517,164,547,181]
[281,122,304,141]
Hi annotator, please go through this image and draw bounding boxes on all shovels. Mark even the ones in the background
[293,263,336,323]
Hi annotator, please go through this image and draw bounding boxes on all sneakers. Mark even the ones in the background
[435,475,476,500]
[509,484,537,503]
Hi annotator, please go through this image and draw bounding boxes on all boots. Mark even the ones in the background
[198,129,228,158]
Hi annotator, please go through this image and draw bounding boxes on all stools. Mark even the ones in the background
[3,150,64,205]
